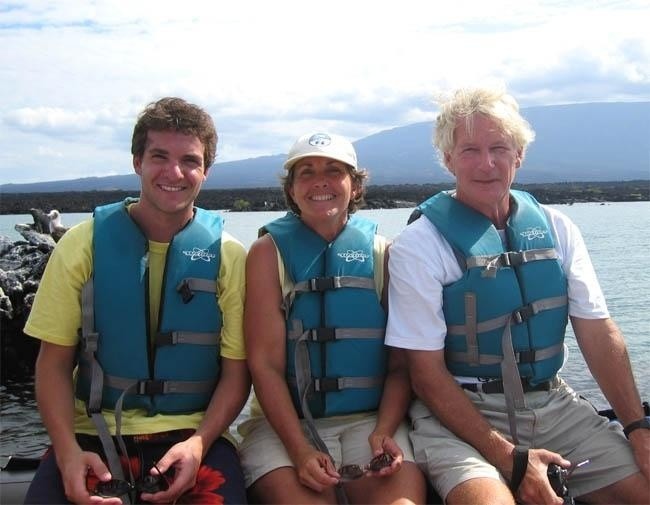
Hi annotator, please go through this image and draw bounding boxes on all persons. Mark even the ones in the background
[236,132,427,505]
[38,210,50,233]
[22,97,248,503]
[384,87,650,505]
[47,205,63,234]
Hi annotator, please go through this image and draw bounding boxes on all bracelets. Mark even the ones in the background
[623,418,650,439]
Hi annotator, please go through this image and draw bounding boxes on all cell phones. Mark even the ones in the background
[547,462,568,497]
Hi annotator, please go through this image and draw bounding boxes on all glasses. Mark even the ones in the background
[335,450,394,479]
[85,460,170,499]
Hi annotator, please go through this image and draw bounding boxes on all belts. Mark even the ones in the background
[461,378,550,393]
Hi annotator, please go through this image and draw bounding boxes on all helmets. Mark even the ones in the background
[283,131,357,171]
